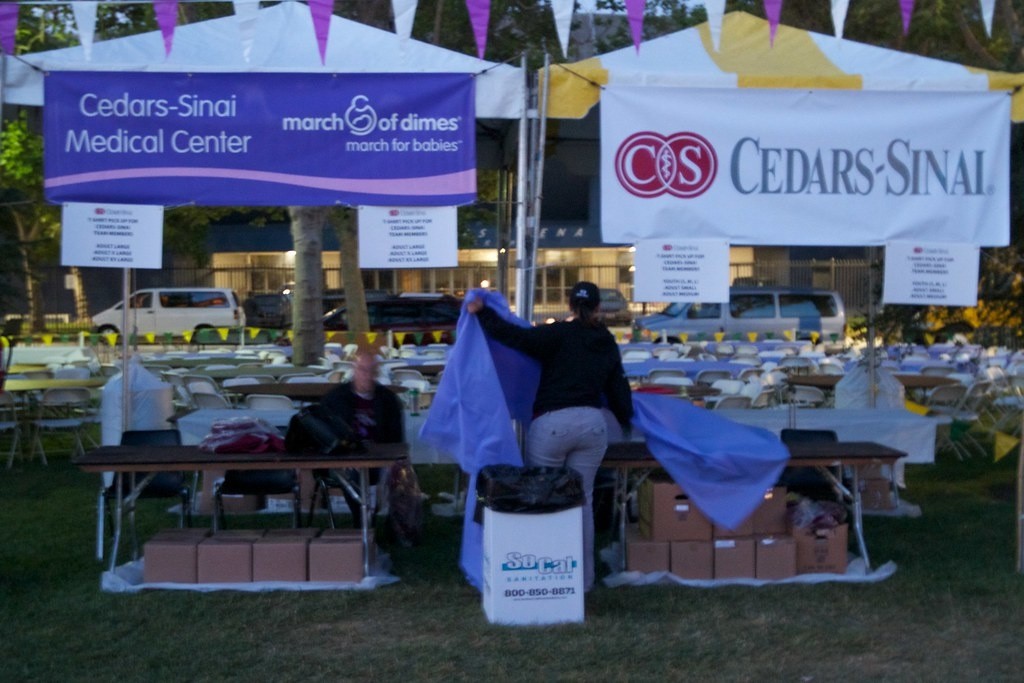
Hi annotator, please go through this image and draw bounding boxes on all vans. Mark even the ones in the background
[91,287,247,343]
[634,287,848,341]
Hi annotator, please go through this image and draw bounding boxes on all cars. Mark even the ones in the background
[595,287,633,327]
[323,288,464,331]
[243,294,291,328]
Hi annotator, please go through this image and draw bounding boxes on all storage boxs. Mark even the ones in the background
[623,468,848,582]
[482,505,585,625]
[142,469,374,583]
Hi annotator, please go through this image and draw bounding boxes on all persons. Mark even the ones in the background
[468,281,635,593]
[316,351,410,530]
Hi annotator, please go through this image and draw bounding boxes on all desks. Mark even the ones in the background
[66,447,406,578]
[622,363,758,376]
[4,377,105,433]
[783,374,959,401]
[6,365,52,376]
[600,441,907,575]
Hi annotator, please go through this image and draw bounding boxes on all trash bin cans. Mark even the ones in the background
[482,463,585,626]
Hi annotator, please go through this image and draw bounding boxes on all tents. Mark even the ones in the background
[3,1,531,431]
[531,13,1024,415]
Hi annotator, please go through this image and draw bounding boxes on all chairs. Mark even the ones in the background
[0,335,1024,470]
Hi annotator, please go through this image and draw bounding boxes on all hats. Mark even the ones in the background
[571,281,601,301]
